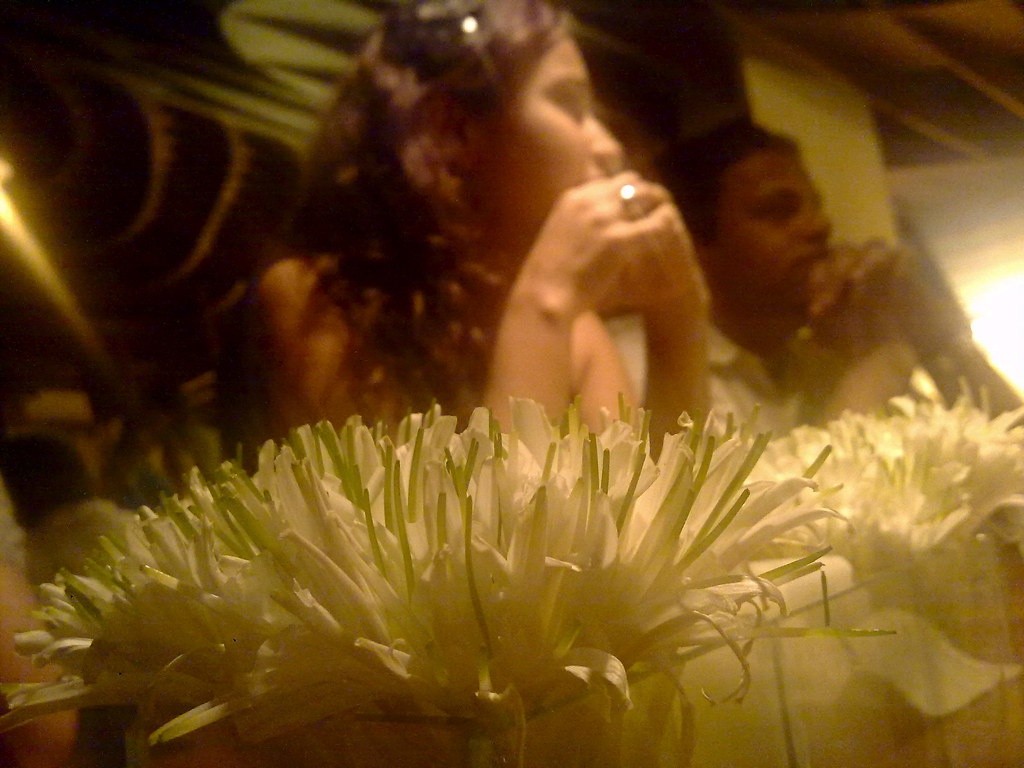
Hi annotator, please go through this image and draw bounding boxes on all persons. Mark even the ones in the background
[223,0,712,488]
[666,120,1024,437]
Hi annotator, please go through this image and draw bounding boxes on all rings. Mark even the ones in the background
[621,186,642,216]
